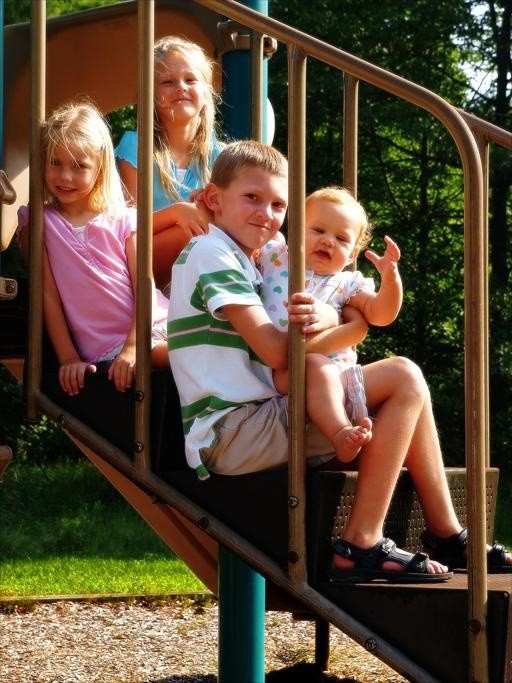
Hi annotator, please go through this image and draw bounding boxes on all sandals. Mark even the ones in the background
[419,529,512,575]
[329,538,454,584]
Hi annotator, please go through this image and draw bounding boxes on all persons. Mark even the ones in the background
[41,99,171,397]
[110,35,228,212]
[191,181,406,463]
[166,140,512,582]
